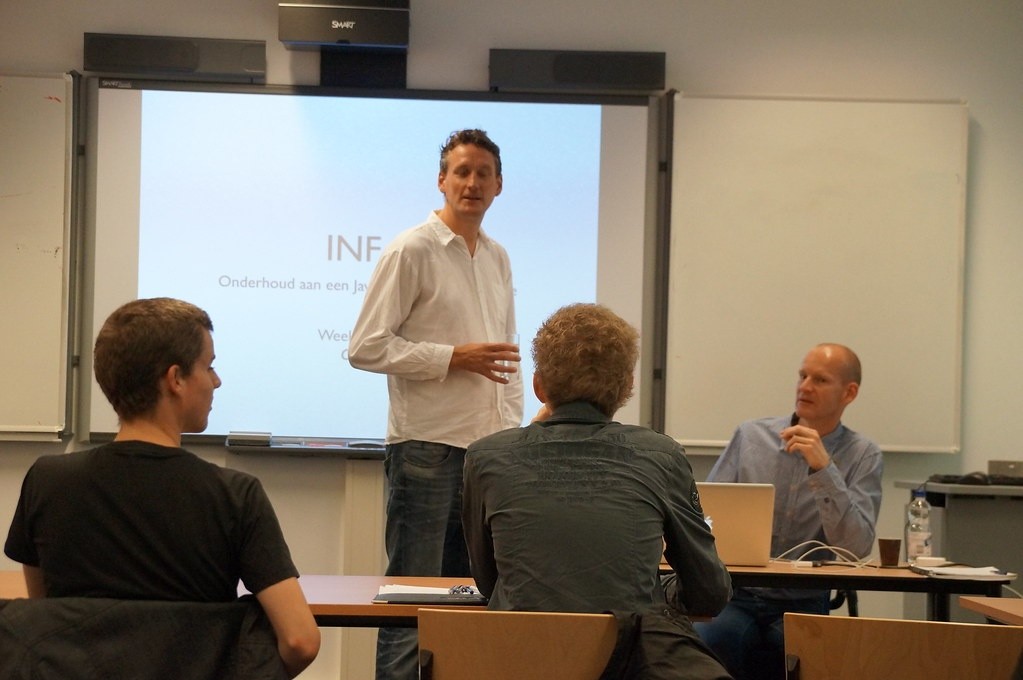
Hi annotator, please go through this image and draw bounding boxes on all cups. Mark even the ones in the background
[491,333,519,383]
[878,537,901,566]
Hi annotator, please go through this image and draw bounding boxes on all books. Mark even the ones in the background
[911,563,1005,577]
[371,583,490,606]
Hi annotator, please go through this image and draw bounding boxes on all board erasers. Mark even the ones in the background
[348,440,384,449]
[227,434,272,446]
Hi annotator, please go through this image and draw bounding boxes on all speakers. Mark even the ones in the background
[488,48,666,90]
[83,32,266,80]
[278,3,410,48]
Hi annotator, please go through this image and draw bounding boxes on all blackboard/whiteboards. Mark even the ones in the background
[0,70,73,441]
[652,89,971,453]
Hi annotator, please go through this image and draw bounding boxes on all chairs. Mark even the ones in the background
[412,606,622,680]
[782,613,1023,680]
[0,597,292,680]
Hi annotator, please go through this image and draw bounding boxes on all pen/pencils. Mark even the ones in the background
[448,585,475,595]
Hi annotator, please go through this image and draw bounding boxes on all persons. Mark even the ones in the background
[347,128,526,680]
[2,297,321,680]
[461,303,732,680]
[689,342,885,680]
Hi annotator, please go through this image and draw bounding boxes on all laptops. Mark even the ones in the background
[660,481,775,566]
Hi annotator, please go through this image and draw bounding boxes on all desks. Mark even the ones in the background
[656,559,1007,594]
[959,596,1023,625]
[1,572,485,629]
[894,479,1022,622]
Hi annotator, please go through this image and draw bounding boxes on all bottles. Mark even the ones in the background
[908,491,931,564]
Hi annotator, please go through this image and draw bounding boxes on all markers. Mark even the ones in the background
[306,442,343,447]
[791,561,822,568]
